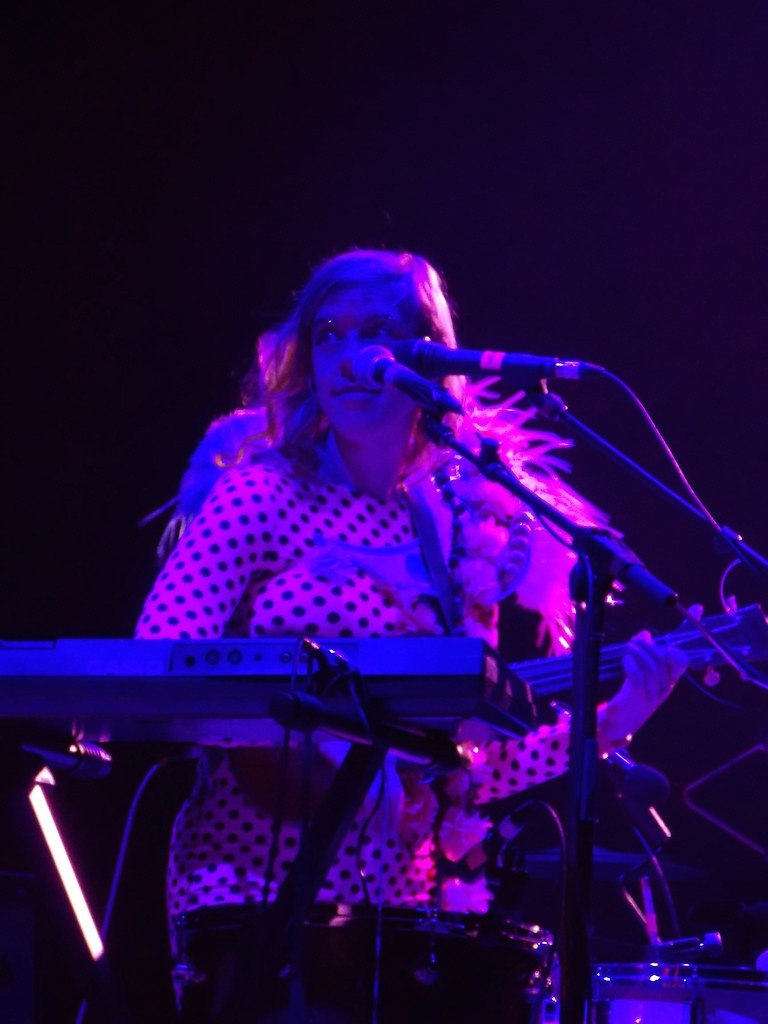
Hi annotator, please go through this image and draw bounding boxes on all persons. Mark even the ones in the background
[139,249,701,1024]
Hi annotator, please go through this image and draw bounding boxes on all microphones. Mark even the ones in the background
[354,345,463,417]
[405,341,583,384]
[548,702,673,804]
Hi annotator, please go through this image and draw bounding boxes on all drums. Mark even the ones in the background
[581,958,768,1024]
[169,899,565,1024]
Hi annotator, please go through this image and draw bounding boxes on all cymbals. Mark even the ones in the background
[523,842,706,881]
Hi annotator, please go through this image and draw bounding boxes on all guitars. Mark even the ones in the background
[221,599,767,829]
